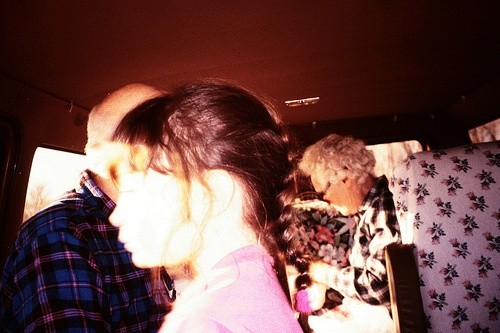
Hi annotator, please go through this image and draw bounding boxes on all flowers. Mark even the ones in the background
[294,198,348,268]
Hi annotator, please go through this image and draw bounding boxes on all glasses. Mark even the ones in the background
[315,173,345,201]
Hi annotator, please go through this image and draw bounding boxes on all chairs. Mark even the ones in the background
[383,140,500,333]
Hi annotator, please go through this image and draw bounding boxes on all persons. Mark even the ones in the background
[298,134,401,305]
[108,79,303,333]
[0,83,165,333]
